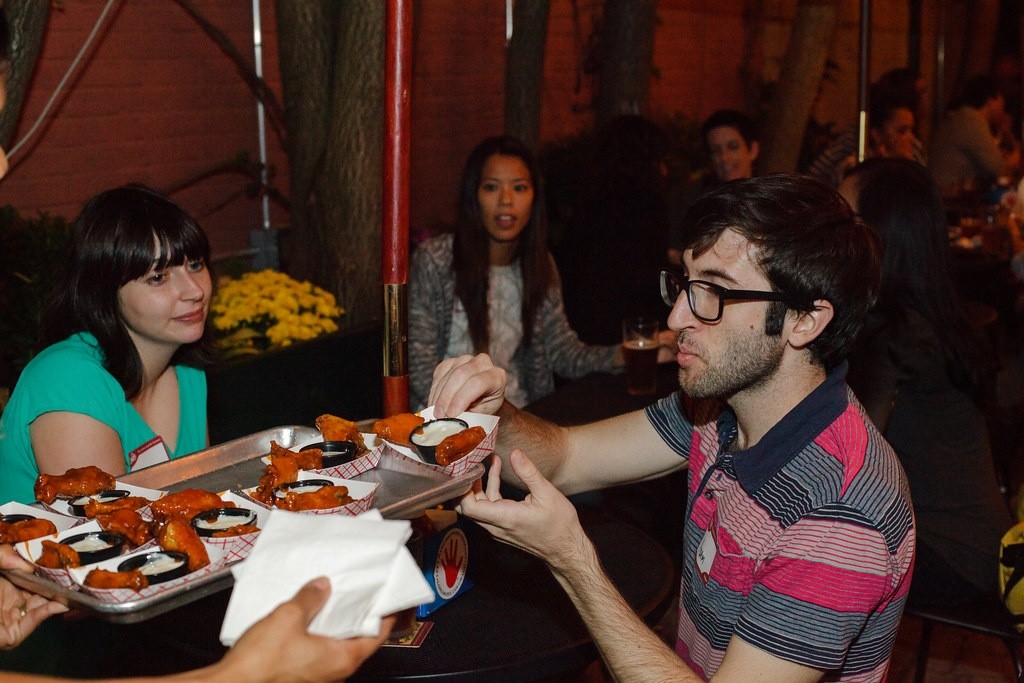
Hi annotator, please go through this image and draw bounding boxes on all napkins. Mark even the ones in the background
[218,509,435,647]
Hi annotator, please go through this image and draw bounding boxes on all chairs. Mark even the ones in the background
[884,384,1024,683]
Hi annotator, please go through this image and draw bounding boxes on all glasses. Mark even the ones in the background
[659,270,816,322]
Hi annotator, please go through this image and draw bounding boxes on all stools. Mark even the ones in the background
[961,300,1003,371]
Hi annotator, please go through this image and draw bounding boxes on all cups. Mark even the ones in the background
[626,319,658,387]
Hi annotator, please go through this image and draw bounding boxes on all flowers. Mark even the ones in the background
[210,270,343,355]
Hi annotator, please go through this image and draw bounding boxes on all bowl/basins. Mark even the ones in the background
[410,418,467,463]
[299,440,357,468]
[117,551,189,586]
[68,491,130,518]
[3,515,35,526]
[192,506,258,536]
[273,478,334,499]
[59,532,122,567]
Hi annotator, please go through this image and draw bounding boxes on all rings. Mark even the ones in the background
[19,604,27,617]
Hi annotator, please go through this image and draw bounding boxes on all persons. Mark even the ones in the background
[557,62,1024,609]
[1,574,397,683]
[424,173,914,683]
[411,138,677,413]
[0,181,212,503]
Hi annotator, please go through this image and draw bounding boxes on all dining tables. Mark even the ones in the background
[13,499,673,682]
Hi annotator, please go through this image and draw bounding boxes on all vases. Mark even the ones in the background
[185,322,350,445]
